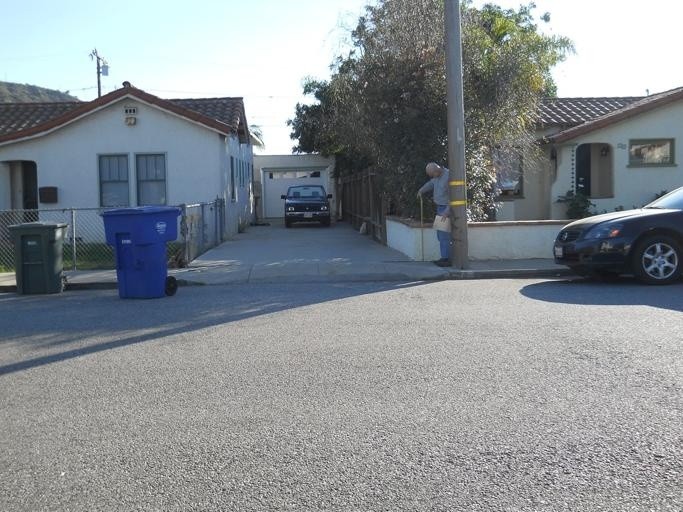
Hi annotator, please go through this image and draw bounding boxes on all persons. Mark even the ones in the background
[417,162,451,267]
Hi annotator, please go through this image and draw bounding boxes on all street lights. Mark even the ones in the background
[95,53,106,95]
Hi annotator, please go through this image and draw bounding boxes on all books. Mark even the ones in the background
[433,215,450,232]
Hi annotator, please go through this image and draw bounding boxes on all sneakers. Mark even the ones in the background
[433,258,451,267]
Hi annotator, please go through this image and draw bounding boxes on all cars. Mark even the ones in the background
[554,186,682,285]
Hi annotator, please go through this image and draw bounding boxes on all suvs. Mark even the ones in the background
[281,185,332,228]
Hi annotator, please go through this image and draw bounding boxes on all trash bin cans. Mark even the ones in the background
[99,206,182,298]
[8,221,69,294]
